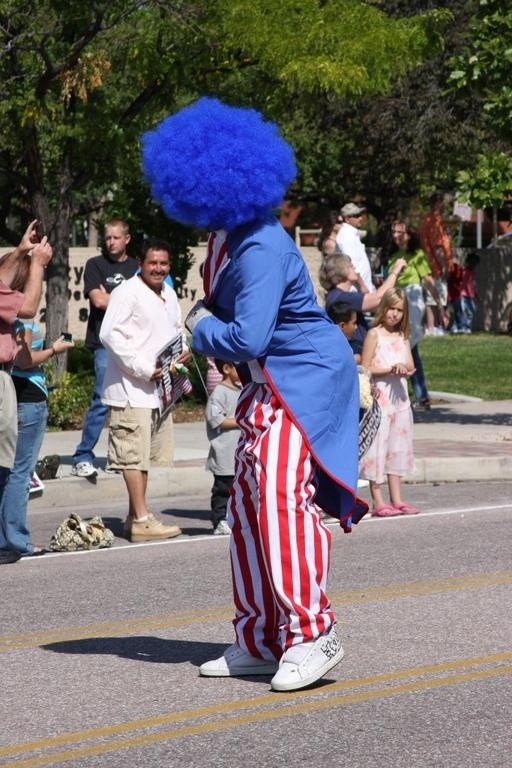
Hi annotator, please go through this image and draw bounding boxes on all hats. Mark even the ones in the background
[340,203,366,217]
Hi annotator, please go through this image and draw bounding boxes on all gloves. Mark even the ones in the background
[186,299,212,334]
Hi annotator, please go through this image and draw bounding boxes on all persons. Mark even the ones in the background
[140,95,369,691]
[70,217,140,476]
[97,240,193,544]
[319,191,481,515]
[0,252,75,556]
[0,218,55,565]
[203,355,245,533]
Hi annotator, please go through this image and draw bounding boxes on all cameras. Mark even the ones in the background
[61,333,72,343]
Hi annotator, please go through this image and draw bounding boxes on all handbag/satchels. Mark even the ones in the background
[35,455,60,480]
[357,366,373,409]
[49,512,114,552]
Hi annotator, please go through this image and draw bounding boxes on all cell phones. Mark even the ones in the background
[35,221,43,242]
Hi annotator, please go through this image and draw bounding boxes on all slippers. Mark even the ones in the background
[394,503,419,514]
[372,505,403,516]
[33,546,45,554]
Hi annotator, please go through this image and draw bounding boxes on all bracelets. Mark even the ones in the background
[49,347,57,359]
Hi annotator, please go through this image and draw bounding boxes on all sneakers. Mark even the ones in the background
[0,550,22,564]
[412,398,430,410]
[213,520,232,535]
[123,513,182,542]
[199,642,279,676]
[270,626,344,690]
[424,327,473,336]
[104,464,123,474]
[71,461,97,477]
